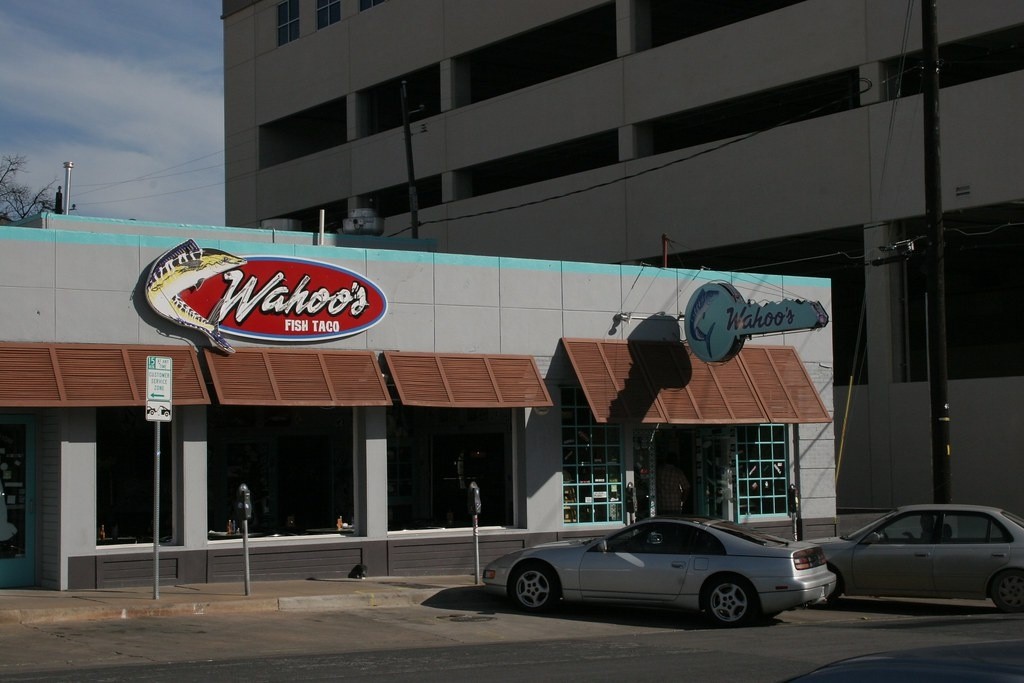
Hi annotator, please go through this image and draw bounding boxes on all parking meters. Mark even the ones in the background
[235,483,254,596]
[465,481,482,585]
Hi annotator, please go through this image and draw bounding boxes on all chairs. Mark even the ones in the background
[943,524,953,539]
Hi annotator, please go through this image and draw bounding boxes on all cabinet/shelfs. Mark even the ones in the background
[388,440,419,516]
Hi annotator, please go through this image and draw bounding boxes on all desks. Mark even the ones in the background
[209,530,265,538]
[305,528,354,534]
[98,537,136,544]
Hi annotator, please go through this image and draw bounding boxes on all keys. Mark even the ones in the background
[684,278,829,365]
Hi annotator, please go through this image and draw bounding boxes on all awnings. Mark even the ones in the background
[383,350,554,407]
[562,337,832,424]
[0,341,211,406]
[202,346,393,405]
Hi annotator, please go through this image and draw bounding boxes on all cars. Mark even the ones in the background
[482,516,836,628]
[794,504,1024,613]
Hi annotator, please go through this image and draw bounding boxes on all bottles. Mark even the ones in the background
[227,520,233,534]
[99,525,105,539]
